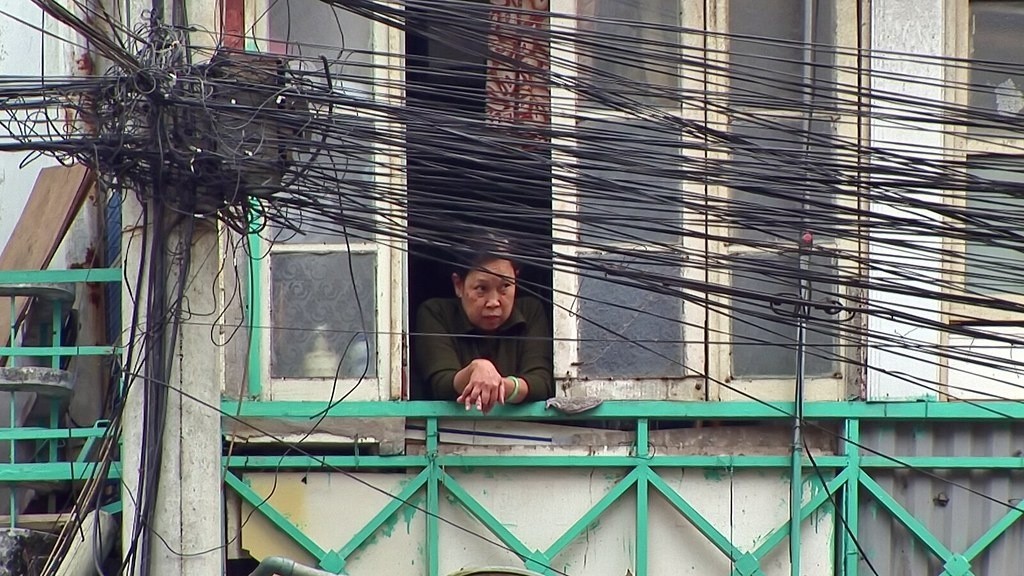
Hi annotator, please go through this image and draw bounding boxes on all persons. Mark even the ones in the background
[412,235,555,415]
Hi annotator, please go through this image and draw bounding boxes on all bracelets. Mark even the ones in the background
[504,375,519,403]
